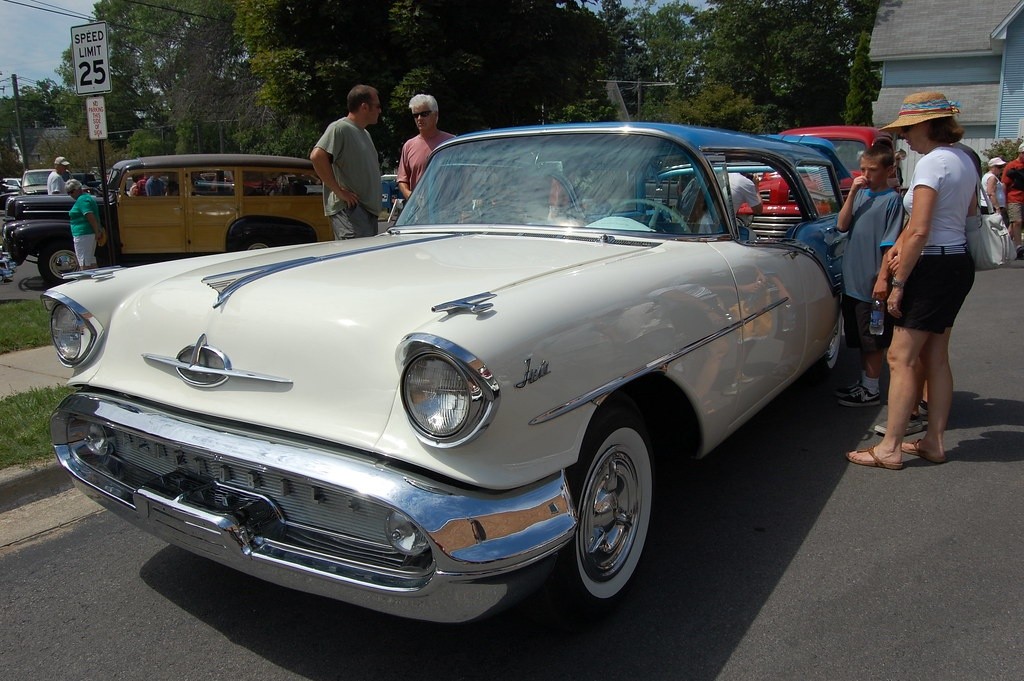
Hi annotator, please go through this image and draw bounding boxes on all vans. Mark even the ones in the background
[105,153,341,255]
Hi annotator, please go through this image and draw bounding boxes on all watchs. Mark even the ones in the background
[889,276,904,287]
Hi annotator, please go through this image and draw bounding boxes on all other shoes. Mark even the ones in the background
[873,415,923,436]
[917,398,930,427]
[1015,246,1024,260]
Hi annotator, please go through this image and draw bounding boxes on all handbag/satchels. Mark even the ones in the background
[965,171,1017,271]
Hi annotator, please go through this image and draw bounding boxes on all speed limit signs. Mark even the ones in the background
[69,21,111,95]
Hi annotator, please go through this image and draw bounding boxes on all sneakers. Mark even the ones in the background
[839,385,881,408]
[835,378,861,396]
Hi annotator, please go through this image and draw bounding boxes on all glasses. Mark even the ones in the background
[995,165,1003,169]
[67,189,75,195]
[902,124,916,133]
[411,110,432,119]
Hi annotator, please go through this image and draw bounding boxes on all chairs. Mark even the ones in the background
[289,183,307,195]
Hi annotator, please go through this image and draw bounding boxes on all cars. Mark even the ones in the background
[40,120,855,628]
[1,159,150,287]
[379,126,900,244]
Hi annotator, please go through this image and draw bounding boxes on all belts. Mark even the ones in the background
[918,242,969,255]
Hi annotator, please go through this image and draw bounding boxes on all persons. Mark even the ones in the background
[846,93,979,469]
[1001,141,1024,259]
[837,145,904,407]
[677,170,763,240]
[648,264,793,406]
[129,174,178,196]
[65,179,101,270]
[310,84,382,241]
[47,156,70,194]
[397,93,457,201]
[979,156,1009,229]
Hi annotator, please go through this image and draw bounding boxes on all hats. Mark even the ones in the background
[988,157,1006,167]
[1018,142,1024,153]
[54,156,70,166]
[877,92,961,133]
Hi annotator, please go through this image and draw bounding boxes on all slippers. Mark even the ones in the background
[901,438,947,463]
[845,445,903,470]
